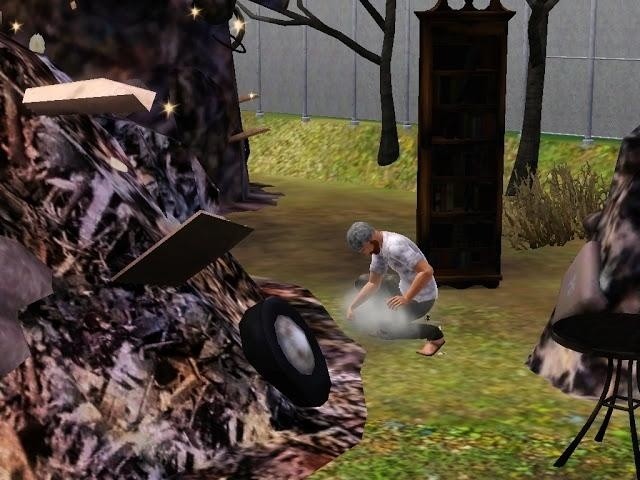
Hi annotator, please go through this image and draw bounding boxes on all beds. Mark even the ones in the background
[552,313,639,480]
